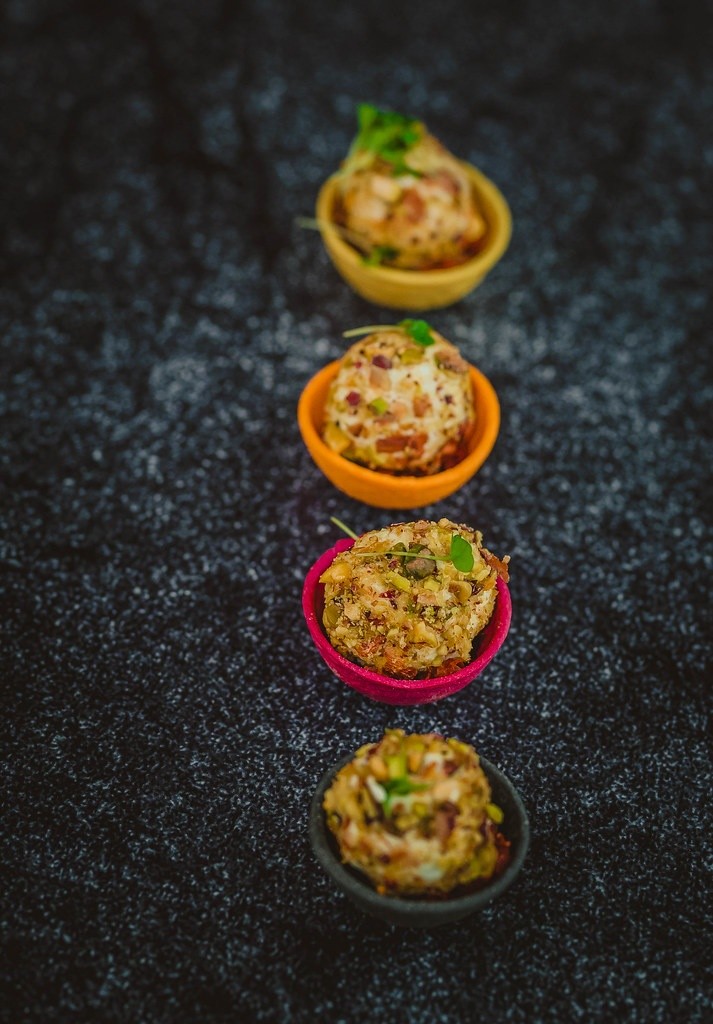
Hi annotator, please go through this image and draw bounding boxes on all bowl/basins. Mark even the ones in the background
[308,753,531,919]
[297,355,500,509]
[306,540,513,704]
[318,162,513,312]
